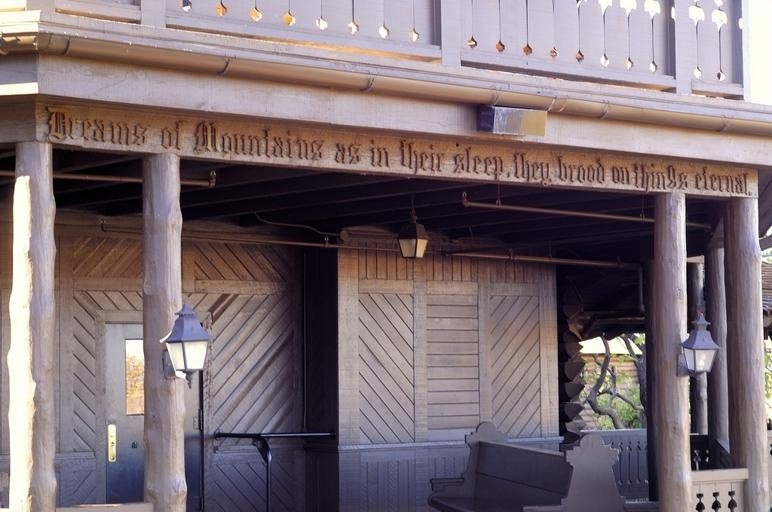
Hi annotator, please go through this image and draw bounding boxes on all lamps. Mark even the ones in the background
[396,195,429,259]
[162,303,211,389]
[676,313,720,377]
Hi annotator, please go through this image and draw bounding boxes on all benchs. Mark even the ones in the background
[428,422,627,512]
